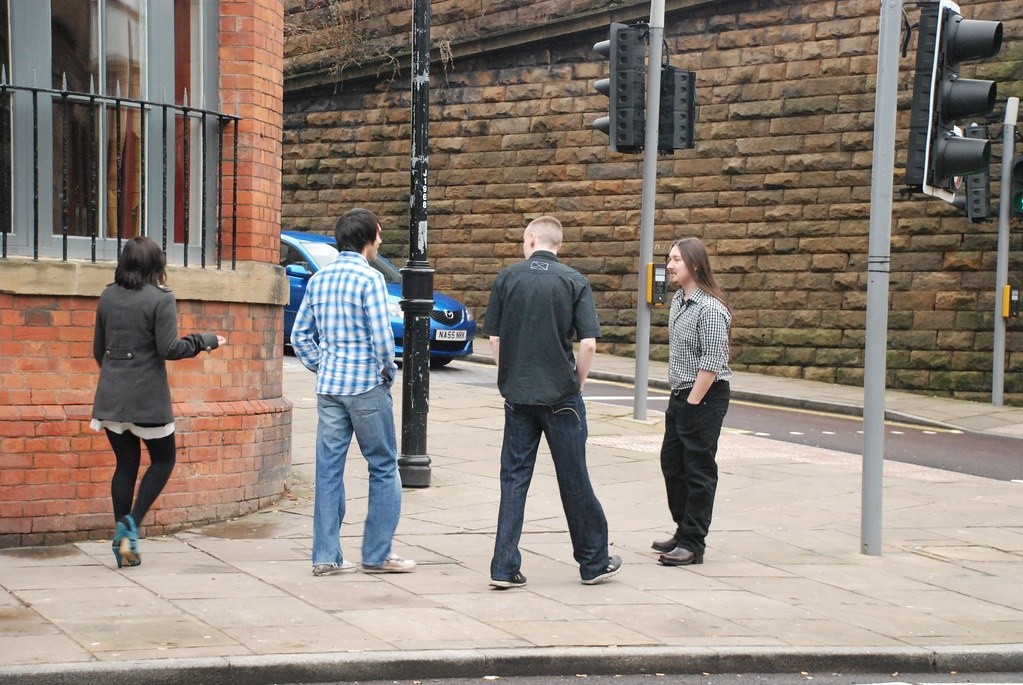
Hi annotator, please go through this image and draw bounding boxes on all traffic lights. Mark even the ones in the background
[1011,156,1023,222]
[922,1,1004,205]
[593,21,643,155]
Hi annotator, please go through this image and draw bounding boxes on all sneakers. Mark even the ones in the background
[581,555,622,584]
[313,561,361,575]
[363,553,415,573]
[490,571,528,587]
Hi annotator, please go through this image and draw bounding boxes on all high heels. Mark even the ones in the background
[111,515,141,568]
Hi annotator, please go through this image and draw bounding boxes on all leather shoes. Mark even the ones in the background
[652,538,678,552]
[659,547,703,564]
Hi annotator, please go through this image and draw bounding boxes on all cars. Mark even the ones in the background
[280,231,476,368]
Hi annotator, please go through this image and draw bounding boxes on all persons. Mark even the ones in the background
[650,235,735,568]
[88,236,227,568]
[482,214,624,589]
[289,208,418,579]
